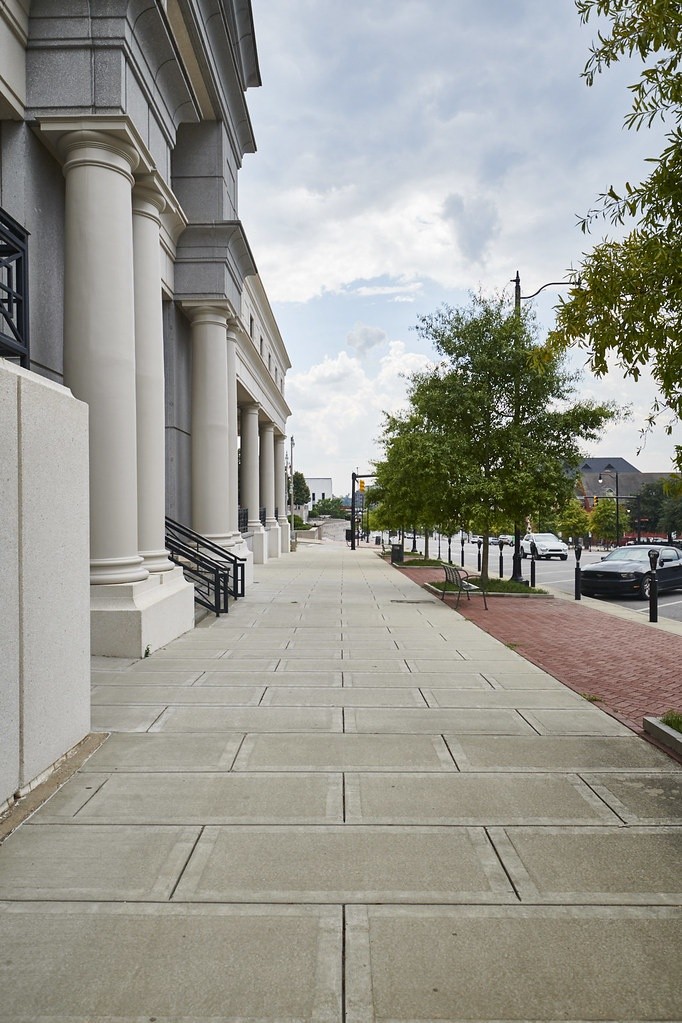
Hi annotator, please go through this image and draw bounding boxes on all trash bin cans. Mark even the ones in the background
[391,544,404,564]
[376,536,380,545]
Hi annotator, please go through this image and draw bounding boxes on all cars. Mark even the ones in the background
[580,545,682,600]
[489,538,500,545]
[403,532,419,538]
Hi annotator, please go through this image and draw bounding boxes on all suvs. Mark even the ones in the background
[471,535,483,544]
[520,533,568,560]
[499,535,513,546]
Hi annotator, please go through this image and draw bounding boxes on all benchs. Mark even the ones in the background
[441,563,489,610]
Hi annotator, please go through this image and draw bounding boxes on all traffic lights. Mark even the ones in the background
[594,496,598,506]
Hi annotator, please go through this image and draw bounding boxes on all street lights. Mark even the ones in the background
[599,471,619,547]
